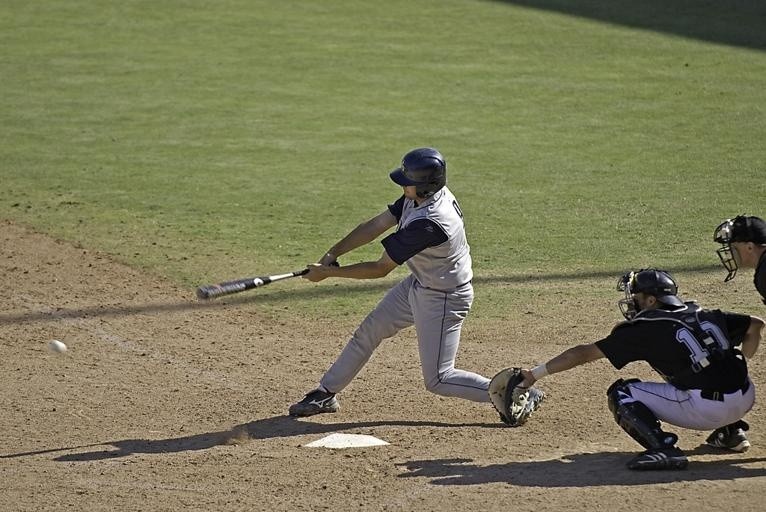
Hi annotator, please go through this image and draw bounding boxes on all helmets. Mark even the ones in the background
[386,148,447,200]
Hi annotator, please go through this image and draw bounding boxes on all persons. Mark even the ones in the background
[714,215,766,302]
[520,268,766,470]
[288,147,547,427]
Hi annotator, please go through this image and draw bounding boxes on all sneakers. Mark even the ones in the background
[628,446,689,474]
[511,386,546,428]
[288,387,341,416]
[706,428,752,453]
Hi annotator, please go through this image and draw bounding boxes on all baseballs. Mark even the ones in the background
[52,340,66,354]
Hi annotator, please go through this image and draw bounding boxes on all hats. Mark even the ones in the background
[633,269,687,310]
[731,216,766,247]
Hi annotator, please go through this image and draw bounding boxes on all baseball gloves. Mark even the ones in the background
[487,367,534,427]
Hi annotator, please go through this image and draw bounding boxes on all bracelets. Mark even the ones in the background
[530,363,548,380]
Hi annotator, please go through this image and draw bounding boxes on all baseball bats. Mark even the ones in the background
[197,263,340,300]
